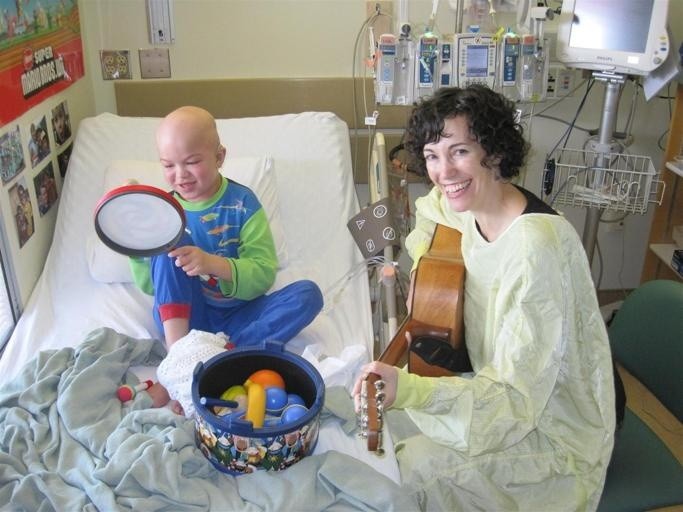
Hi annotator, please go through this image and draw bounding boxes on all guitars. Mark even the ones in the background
[359,224,465,459]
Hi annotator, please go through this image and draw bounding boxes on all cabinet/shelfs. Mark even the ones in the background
[639,82,683,287]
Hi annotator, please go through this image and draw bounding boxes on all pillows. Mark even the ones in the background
[87,158,290,284]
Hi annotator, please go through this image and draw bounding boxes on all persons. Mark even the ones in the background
[14,101,74,248]
[116,106,323,416]
[350,84,616,512]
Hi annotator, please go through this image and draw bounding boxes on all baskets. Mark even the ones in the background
[543,148,665,214]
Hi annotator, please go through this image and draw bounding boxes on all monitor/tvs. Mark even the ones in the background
[554,0,670,75]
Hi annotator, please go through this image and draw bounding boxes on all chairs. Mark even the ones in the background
[596,279,683,512]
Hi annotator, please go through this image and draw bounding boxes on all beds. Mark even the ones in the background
[0,112,396,512]
